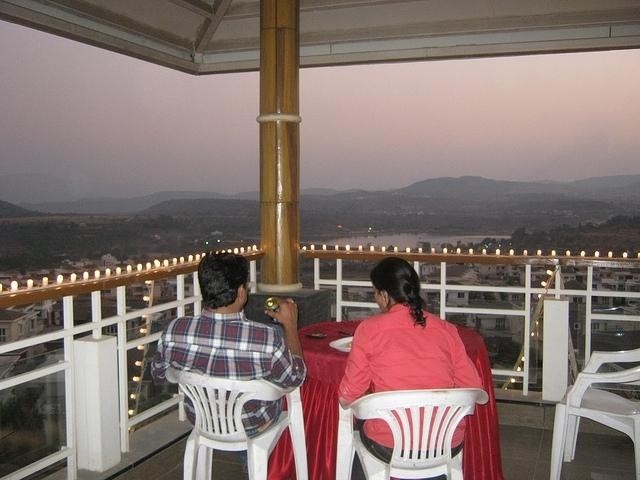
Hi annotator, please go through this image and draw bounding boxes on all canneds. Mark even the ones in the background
[264,296,281,323]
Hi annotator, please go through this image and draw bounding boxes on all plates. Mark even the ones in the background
[328,335,354,353]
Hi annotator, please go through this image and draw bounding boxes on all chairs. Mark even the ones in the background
[551,348,640,480]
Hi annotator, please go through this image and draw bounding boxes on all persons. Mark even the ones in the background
[150,253,308,474]
[338,257,483,480]
